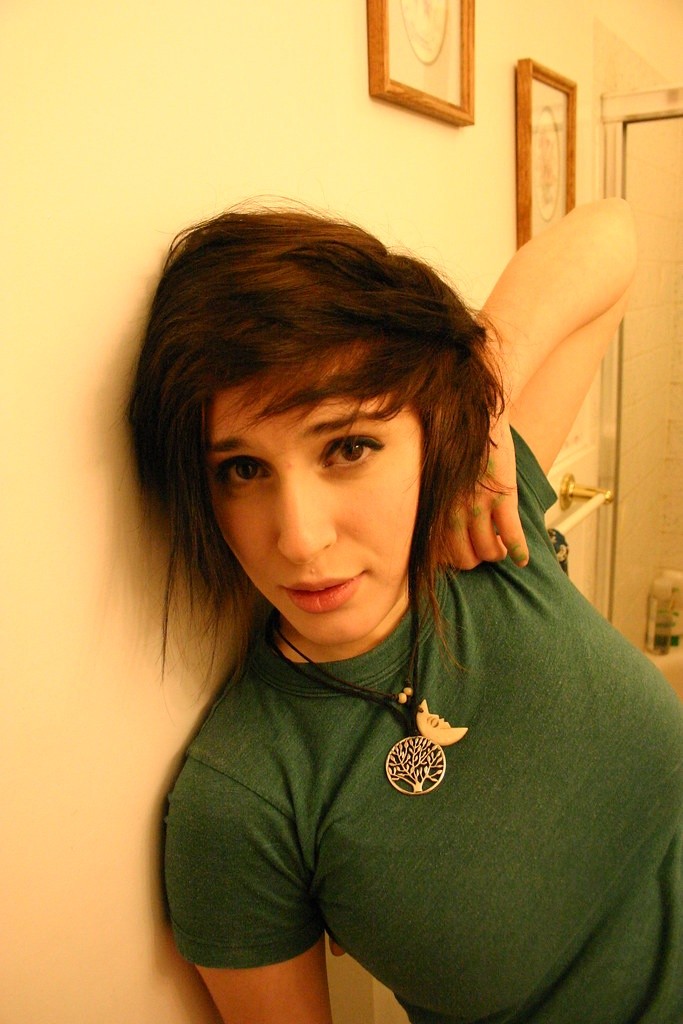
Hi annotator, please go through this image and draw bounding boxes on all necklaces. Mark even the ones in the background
[261,566,470,797]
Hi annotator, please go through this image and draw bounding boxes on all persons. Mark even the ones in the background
[125,198,683,1024]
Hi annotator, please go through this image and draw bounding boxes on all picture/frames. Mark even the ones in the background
[366,0,475,128]
[516,57,576,252]
